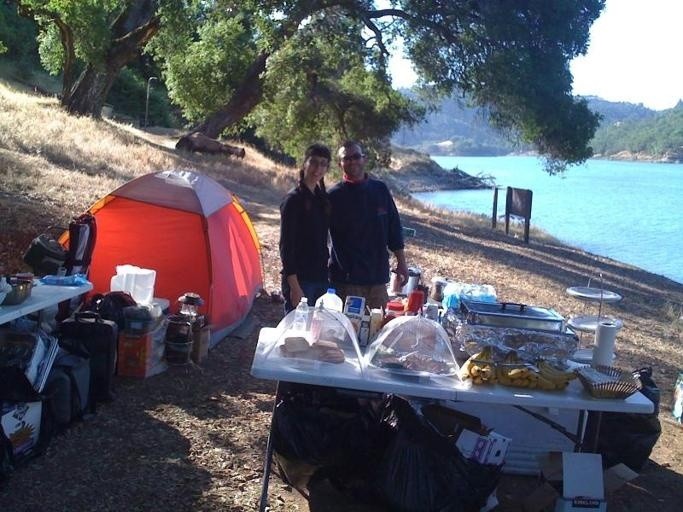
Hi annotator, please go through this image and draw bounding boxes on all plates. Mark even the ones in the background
[367,354,457,382]
[565,314,624,332]
[566,286,622,302]
[276,340,346,373]
[572,349,617,362]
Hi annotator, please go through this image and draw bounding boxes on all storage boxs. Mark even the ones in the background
[454,426,640,512]
[117,319,169,378]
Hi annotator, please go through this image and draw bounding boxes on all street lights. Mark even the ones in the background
[145,76,157,130]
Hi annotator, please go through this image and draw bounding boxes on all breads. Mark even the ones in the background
[280,336,344,363]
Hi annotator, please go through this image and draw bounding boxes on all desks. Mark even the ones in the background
[0,279,94,334]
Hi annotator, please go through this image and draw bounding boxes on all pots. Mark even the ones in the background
[0,279,37,306]
[461,299,566,335]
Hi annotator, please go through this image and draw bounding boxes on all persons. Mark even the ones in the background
[279,141,410,317]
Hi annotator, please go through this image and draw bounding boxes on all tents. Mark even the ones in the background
[56,170,265,352]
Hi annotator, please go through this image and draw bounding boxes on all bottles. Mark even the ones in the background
[384,302,406,319]
[290,297,309,340]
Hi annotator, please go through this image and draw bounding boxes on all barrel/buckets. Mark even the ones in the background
[310,287,342,339]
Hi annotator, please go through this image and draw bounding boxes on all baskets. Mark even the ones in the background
[573,364,642,399]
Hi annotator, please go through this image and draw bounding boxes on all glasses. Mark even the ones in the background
[339,154,365,162]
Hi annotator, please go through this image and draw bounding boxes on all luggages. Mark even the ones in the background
[0,291,136,478]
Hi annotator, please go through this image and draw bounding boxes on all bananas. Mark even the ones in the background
[460,346,577,391]
